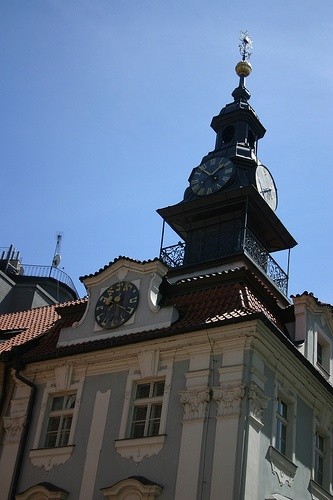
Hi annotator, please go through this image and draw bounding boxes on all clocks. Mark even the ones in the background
[93,281,140,330]
[189,156,235,197]
[254,165,279,212]
